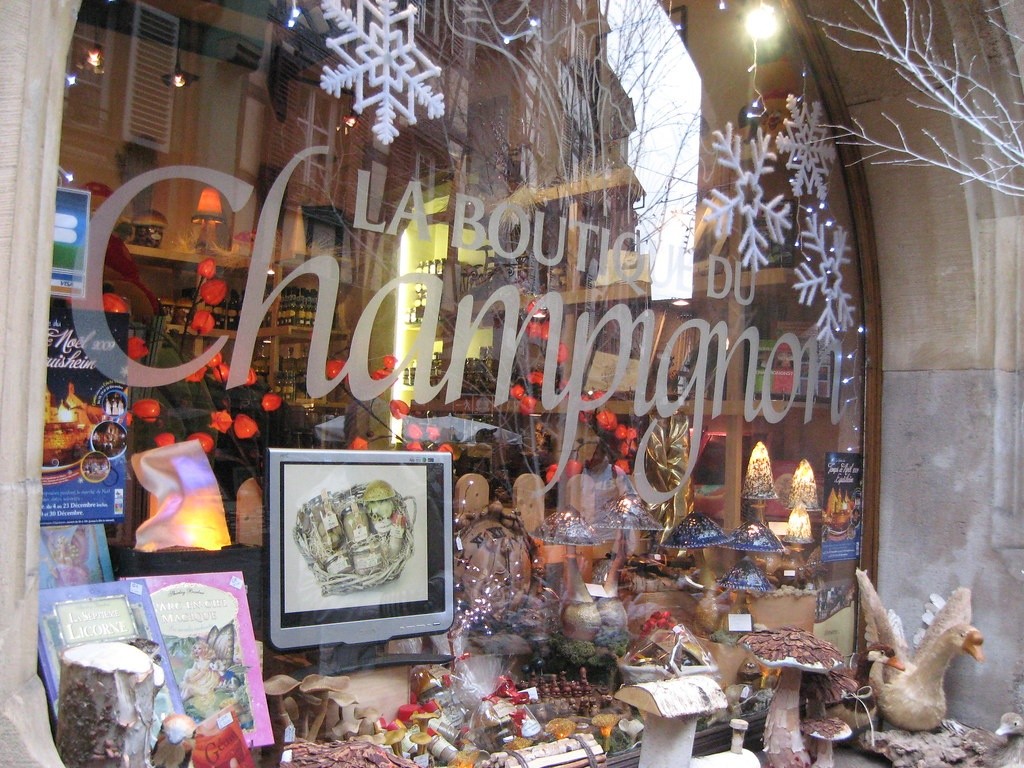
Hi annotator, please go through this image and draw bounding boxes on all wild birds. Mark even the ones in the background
[942,694,1024,768]
[825,643,904,749]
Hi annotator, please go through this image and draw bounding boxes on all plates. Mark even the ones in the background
[851,518,860,527]
[42,459,80,474]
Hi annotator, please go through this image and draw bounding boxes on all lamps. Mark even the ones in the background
[157,50,200,90]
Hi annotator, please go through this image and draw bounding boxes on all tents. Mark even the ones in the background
[312,413,525,449]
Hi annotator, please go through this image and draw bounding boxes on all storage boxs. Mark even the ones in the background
[43,307,275,749]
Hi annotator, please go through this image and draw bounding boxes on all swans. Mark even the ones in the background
[856,568,982,733]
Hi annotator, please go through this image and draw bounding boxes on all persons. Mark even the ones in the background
[579,434,635,534]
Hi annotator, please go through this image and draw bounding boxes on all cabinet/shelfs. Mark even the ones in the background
[405,145,860,613]
[108,242,370,448]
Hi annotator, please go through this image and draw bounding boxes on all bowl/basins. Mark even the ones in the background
[828,527,849,536]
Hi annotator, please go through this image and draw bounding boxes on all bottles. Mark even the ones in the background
[409,665,466,728]
[854,488,861,510]
[843,489,850,514]
[827,488,835,517]
[836,487,842,513]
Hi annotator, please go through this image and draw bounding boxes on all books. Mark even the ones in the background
[37,522,116,590]
[34,570,276,751]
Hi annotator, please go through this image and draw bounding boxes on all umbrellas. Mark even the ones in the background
[579,350,644,395]
